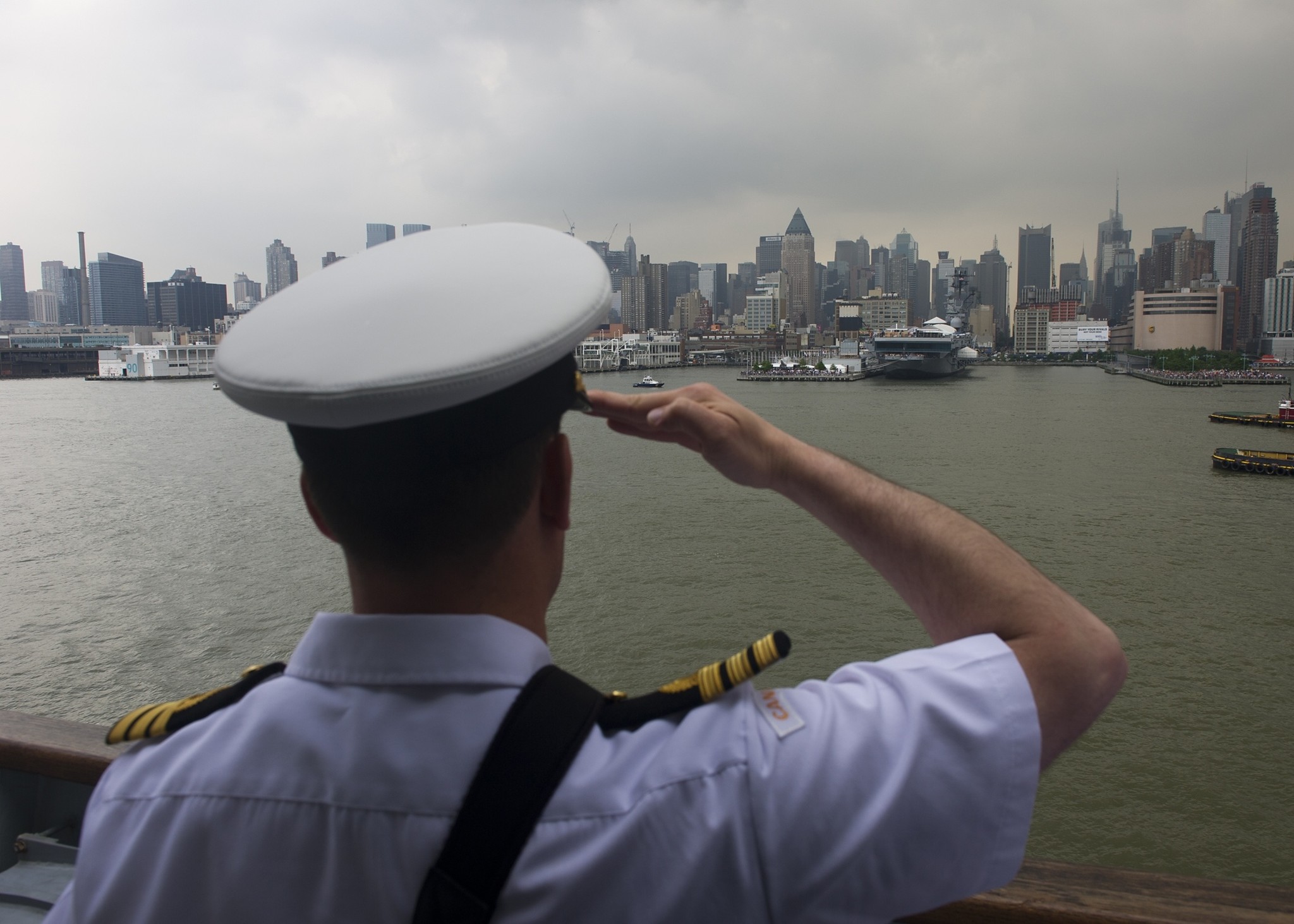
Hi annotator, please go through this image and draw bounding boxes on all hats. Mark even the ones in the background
[213,222,614,463]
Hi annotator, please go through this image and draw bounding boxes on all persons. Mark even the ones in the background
[45,221,1126,924]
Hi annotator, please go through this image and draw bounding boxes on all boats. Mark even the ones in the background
[865,267,989,380]
[212,382,220,390]
[1208,384,1294,429]
[633,374,665,388]
[1212,447,1294,476]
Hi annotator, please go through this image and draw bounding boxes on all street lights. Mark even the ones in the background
[1240,358,1249,371]
[1145,355,1153,370]
[1189,358,1198,372]
[1159,357,1168,370]
